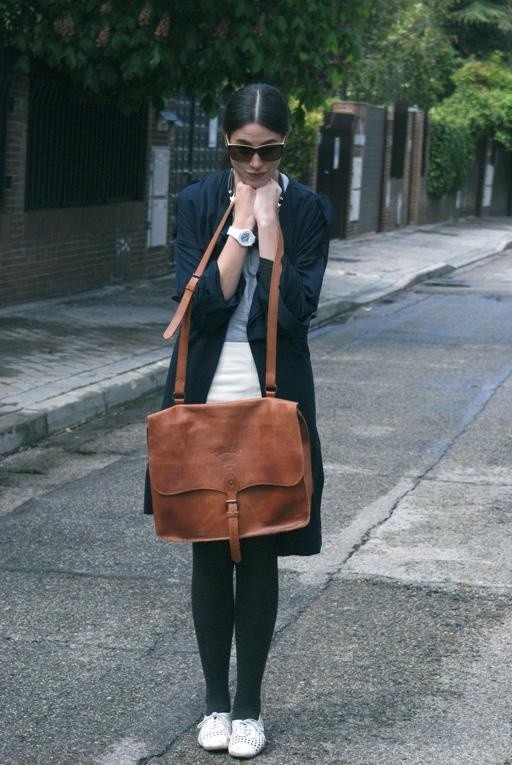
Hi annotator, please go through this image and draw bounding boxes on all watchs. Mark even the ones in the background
[226,225,257,247]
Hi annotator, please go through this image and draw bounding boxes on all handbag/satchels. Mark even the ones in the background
[145,196,313,565]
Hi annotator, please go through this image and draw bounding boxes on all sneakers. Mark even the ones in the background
[227,711,266,758]
[196,711,231,751]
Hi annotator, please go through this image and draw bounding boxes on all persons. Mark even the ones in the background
[144,82,332,759]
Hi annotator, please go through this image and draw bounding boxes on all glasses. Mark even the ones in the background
[224,136,287,163]
